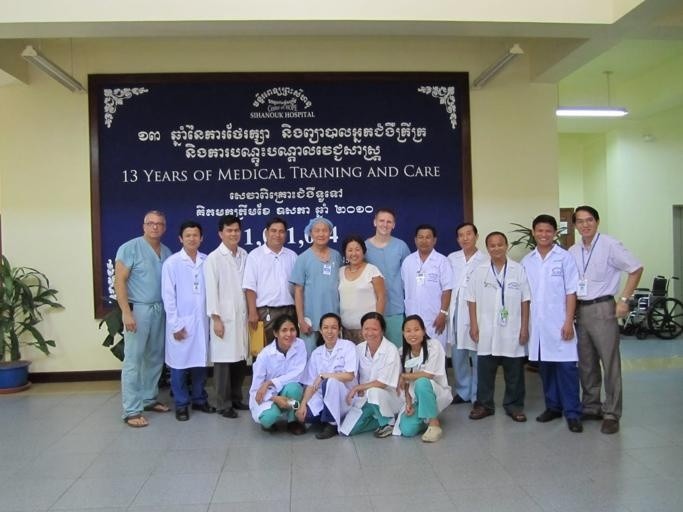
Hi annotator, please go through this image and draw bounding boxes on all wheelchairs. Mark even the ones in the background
[618,270,683,340]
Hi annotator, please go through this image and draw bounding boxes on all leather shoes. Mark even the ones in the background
[452,395,619,433]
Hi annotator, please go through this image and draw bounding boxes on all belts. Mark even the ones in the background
[577,295,613,304]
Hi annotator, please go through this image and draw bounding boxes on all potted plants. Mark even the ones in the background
[0,254,65,390]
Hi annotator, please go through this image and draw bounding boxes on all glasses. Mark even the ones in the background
[144,221,164,226]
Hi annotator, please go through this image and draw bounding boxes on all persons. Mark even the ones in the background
[248,312,453,443]
[204,215,250,418]
[114,210,216,427]
[399,205,644,435]
[242,206,412,385]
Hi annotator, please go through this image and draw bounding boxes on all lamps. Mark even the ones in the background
[21,39,86,92]
[473,42,524,89]
[555,71,629,116]
